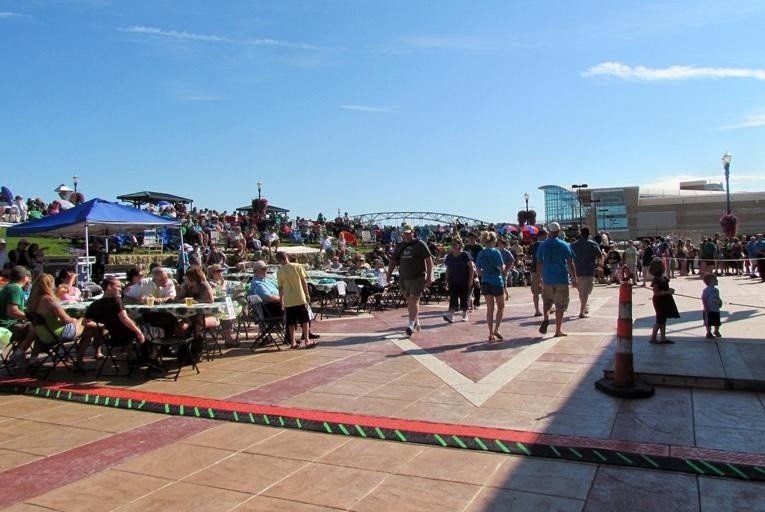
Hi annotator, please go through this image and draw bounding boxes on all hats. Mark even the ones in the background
[209,263,227,272]
[548,222,562,232]
[402,223,414,234]
[253,260,269,271]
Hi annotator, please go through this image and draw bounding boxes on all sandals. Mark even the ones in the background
[579,313,590,318]
[305,340,320,349]
[538,319,550,334]
[648,338,676,345]
[291,343,300,349]
[225,340,242,348]
[554,330,567,337]
[535,308,556,316]
[583,307,589,314]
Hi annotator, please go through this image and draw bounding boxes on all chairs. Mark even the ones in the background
[143,238,163,255]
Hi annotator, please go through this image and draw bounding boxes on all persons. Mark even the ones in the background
[0,194,765,377]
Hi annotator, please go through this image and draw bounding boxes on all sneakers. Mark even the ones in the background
[10,352,30,371]
[460,314,468,321]
[489,329,504,343]
[128,358,161,374]
[443,314,455,323]
[412,325,422,333]
[468,305,480,313]
[406,326,413,336]
[284,335,301,345]
[301,332,320,340]
[706,331,721,338]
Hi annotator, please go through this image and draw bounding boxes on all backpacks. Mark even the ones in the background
[178,332,203,365]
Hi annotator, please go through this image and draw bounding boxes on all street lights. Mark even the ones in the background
[72,175,79,206]
[572,184,588,233]
[598,208,609,231]
[589,200,600,237]
[610,218,615,228]
[721,151,733,238]
[613,223,619,228]
[524,192,530,225]
[256,179,263,199]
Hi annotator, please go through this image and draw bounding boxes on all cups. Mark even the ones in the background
[185,297,193,307]
[146,297,155,307]
